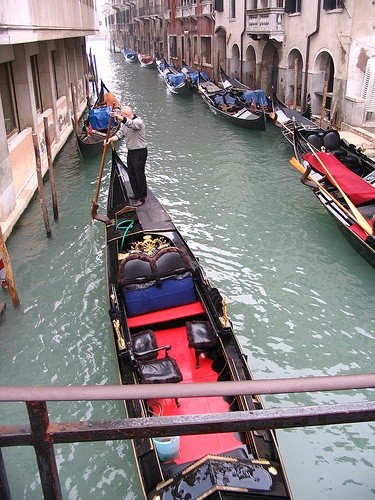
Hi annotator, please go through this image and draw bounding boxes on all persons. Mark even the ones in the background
[103,106,148,207]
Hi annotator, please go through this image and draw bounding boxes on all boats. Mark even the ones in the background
[122,46,138,63]
[75,78,122,146]
[269,85,327,146]
[156,51,179,74]
[161,56,189,95]
[102,138,295,500]
[218,63,310,122]
[197,70,266,132]
[137,46,155,69]
[290,115,375,272]
[180,55,209,88]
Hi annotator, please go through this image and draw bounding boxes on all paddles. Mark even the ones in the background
[288,156,375,241]
[307,142,374,236]
[89,100,116,221]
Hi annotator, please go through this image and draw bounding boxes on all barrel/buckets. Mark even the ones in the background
[151,436,180,462]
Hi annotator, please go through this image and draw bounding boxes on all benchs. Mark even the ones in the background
[132,331,156,359]
[187,321,215,369]
[306,129,346,157]
[167,447,249,478]
[119,247,205,329]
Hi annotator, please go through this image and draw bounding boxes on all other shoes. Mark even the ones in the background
[128,194,146,207]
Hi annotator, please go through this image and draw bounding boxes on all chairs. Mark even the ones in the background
[128,345,182,408]
